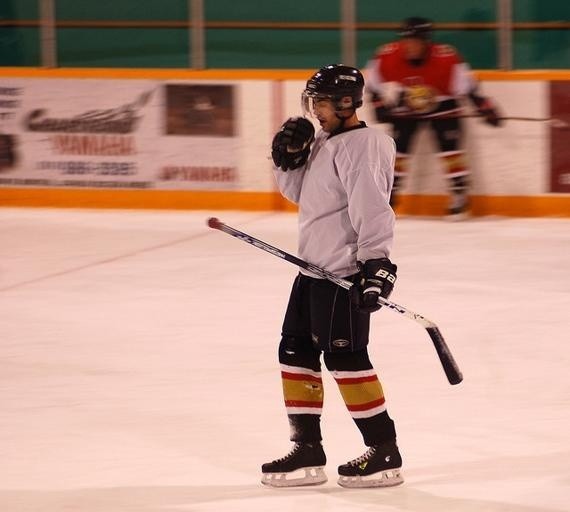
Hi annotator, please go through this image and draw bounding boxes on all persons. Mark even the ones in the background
[260,63,406,488]
[364,16,506,220]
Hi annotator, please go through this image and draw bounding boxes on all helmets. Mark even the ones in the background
[300,65,365,122]
[396,17,433,39]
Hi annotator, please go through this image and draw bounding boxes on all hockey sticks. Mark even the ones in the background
[411,117,569,129]
[208,217,463,385]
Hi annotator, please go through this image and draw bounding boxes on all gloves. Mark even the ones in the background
[468,94,502,126]
[271,116,315,171]
[348,257,398,316]
[371,93,394,124]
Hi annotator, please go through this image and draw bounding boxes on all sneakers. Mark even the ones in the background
[338,445,402,476]
[449,193,469,215]
[261,440,327,472]
